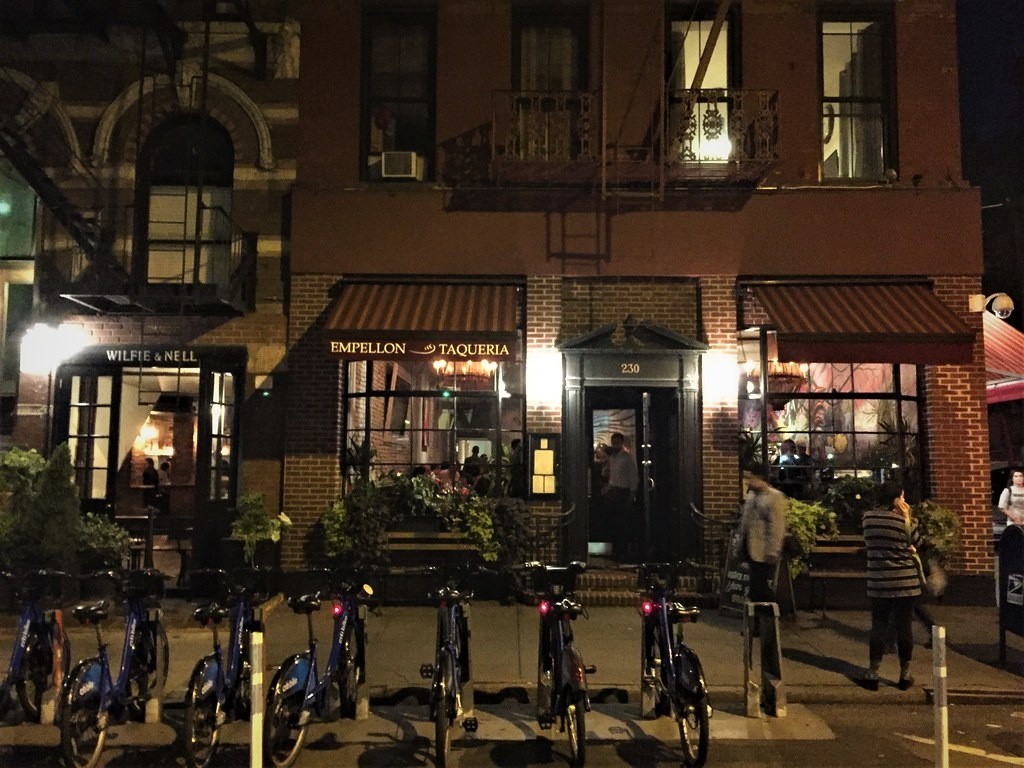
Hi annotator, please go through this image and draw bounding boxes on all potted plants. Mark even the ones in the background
[909,501,958,598]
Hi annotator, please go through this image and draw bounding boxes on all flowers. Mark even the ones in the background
[229,495,291,544]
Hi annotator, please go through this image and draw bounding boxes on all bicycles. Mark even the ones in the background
[0,569,72,721]
[609,557,718,768]
[404,560,499,768]
[262,568,381,768]
[182,568,279,768]
[53,567,175,768]
[512,560,603,765]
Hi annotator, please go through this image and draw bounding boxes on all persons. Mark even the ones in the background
[997,469,1024,526]
[414,438,525,499]
[143,451,228,511]
[592,433,639,565]
[738,440,817,636]
[852,479,938,691]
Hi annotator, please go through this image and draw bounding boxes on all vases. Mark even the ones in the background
[223,538,278,602]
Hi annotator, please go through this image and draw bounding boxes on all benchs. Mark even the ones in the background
[378,532,479,575]
[806,534,868,618]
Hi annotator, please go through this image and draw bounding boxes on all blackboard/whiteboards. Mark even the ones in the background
[718,528,797,616]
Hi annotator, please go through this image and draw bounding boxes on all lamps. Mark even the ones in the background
[753,352,805,410]
[434,359,498,390]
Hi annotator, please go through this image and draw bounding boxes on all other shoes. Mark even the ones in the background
[899,675,914,690]
[922,637,934,647]
[740,624,760,637]
[883,641,897,655]
[857,676,879,691]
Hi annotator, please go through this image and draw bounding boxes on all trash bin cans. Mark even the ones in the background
[999,524,1024,667]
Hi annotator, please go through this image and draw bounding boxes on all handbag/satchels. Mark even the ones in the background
[921,558,949,597]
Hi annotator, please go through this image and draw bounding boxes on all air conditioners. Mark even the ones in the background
[382,152,417,177]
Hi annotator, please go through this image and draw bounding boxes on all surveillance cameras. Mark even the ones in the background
[991,295,1014,319]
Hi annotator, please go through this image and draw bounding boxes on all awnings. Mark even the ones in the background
[322,279,518,366]
[746,285,975,365]
[982,311,1024,403]
